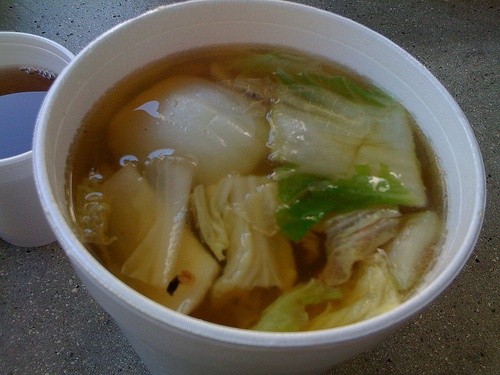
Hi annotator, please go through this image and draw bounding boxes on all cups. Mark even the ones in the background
[1,30,76,247]
[33,0,486,375]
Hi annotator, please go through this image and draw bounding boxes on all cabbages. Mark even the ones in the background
[77,46,442,331]
